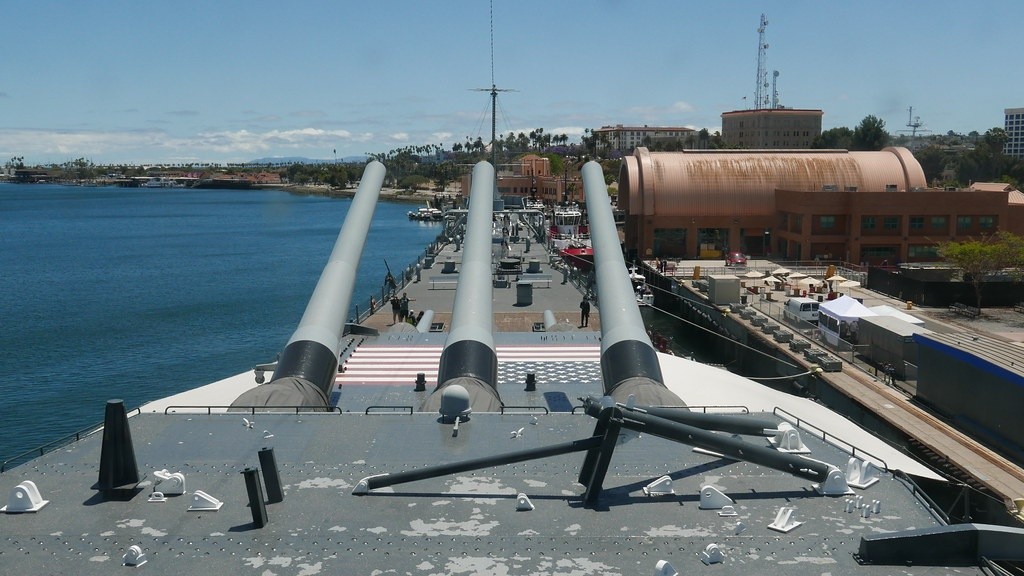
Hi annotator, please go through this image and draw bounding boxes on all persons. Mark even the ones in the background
[390,292,416,327]
[879,361,896,383]
[581,294,591,326]
[822,280,827,293]
[561,263,594,288]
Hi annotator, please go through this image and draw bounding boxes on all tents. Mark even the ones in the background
[816,295,878,348]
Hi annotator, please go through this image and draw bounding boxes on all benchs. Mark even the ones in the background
[518,274,552,288]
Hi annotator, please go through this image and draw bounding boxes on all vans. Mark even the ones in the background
[783,298,819,327]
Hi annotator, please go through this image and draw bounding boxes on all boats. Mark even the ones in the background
[542,168,655,306]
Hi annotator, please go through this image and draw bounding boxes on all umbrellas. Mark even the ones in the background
[745,267,860,298]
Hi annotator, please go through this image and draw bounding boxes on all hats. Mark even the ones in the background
[410,310,413,313]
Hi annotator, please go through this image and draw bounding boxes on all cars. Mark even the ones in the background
[725,251,749,267]
